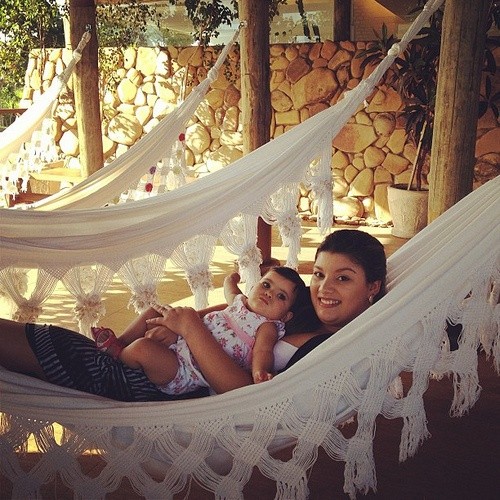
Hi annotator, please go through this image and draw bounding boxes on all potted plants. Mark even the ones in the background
[355,0,500,239]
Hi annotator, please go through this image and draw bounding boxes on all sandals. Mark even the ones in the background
[90,326,121,362]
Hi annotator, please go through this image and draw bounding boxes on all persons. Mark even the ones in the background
[0,229,387,402]
[90,267,307,394]
